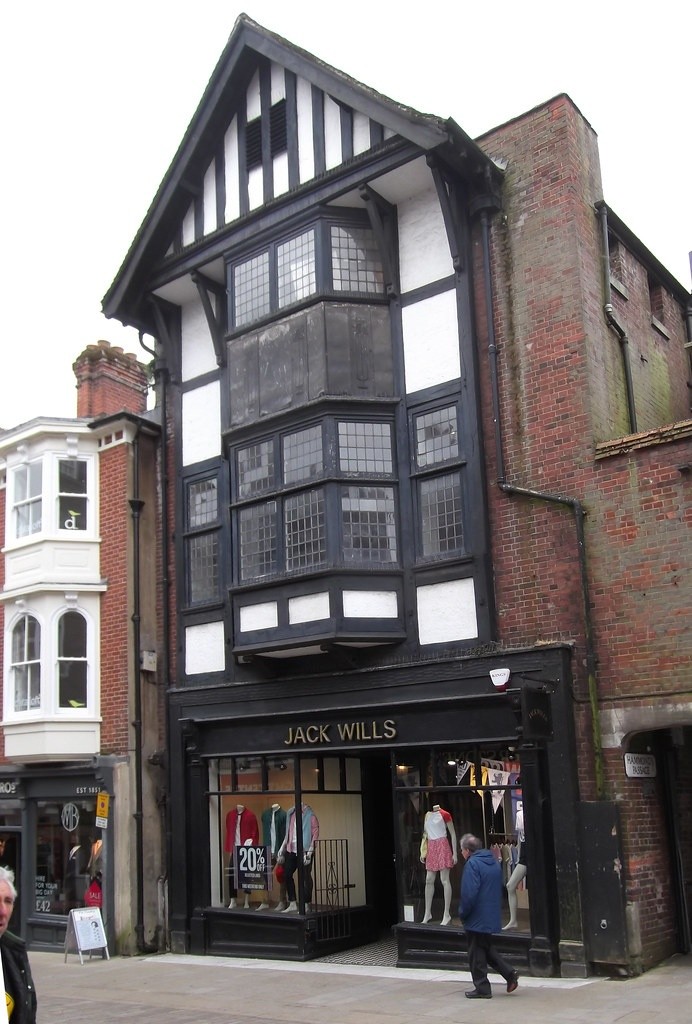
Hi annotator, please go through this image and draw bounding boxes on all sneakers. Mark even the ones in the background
[506,969,520,992]
[465,988,491,998]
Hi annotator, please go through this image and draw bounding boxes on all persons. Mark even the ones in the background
[224,803,257,909]
[419,805,458,925]
[85,839,103,904]
[501,837,528,933]
[457,832,520,1000]
[277,801,320,914]
[254,802,287,911]
[0,865,37,1024]
